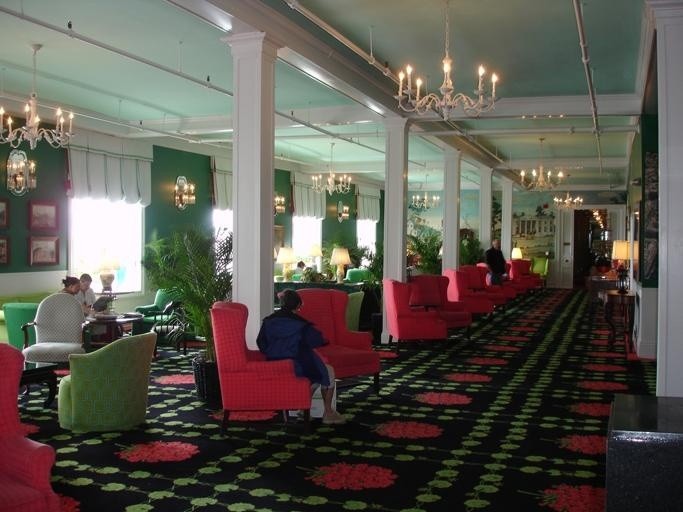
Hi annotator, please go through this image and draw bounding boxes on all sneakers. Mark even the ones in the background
[323,415,346,424]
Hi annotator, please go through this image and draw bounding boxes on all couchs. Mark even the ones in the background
[58,330,159,433]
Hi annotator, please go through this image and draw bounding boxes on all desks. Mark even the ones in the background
[84,313,144,350]
[604,289,636,333]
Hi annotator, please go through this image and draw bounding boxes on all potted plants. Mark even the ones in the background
[141,226,234,401]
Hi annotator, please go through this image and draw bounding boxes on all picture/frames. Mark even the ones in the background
[29,236,60,266]
[0,198,10,230]
[28,202,58,232]
[0,235,11,267]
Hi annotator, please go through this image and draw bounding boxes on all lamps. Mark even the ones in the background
[553,192,583,210]
[8,151,37,196]
[412,173,440,210]
[520,136,565,191]
[612,240,630,278]
[0,44,75,150]
[393,1,498,123]
[512,247,522,259]
[312,142,351,195]
[330,248,352,284]
[174,176,195,211]
[98,251,120,291]
[275,247,296,282]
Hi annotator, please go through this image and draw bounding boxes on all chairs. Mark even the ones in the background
[0,343,61,512]
[21,293,89,395]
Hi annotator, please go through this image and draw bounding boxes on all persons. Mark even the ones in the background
[73,274,96,318]
[57,275,80,297]
[484,238,506,285]
[294,261,306,275]
[256,288,347,423]
